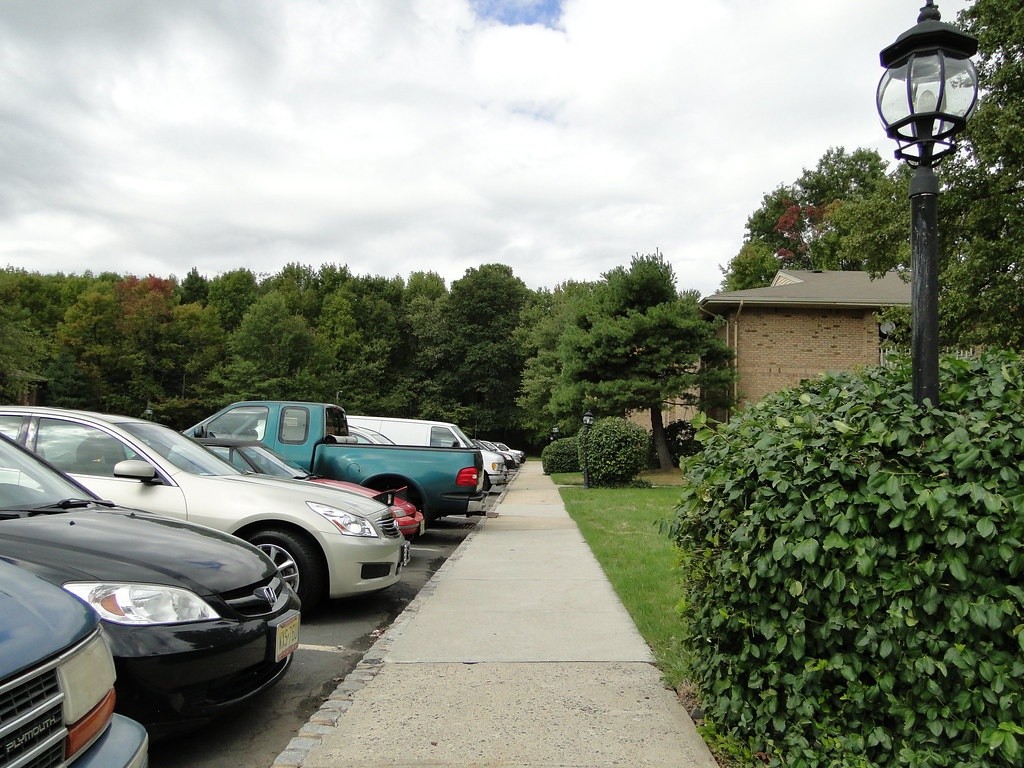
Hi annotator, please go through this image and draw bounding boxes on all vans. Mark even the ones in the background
[346,414,509,491]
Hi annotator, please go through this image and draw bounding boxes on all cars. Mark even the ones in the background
[1,405,412,625]
[188,425,426,537]
[0,432,302,732]
[347,425,395,445]
[470,439,526,469]
[0,558,150,768]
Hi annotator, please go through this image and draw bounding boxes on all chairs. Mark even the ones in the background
[75,436,116,477]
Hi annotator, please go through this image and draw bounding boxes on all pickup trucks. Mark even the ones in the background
[181,400,490,529]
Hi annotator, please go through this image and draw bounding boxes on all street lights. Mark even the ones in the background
[874,0,980,414]
[581,407,593,489]
